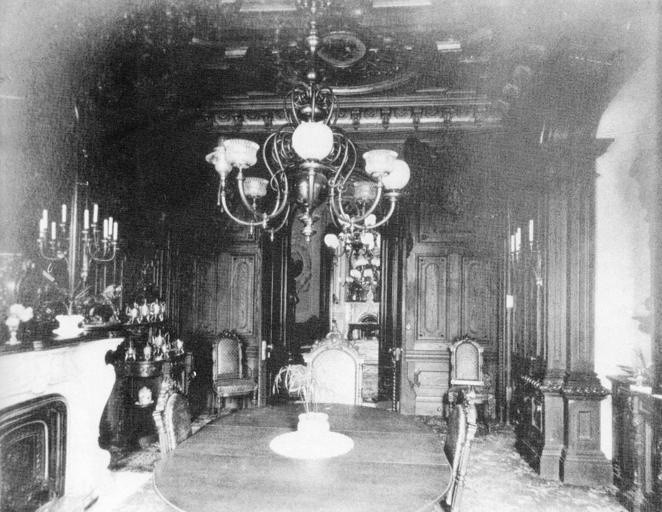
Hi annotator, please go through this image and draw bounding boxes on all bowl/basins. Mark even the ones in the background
[297,411,329,442]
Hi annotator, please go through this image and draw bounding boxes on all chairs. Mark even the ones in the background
[306,327,364,405]
[211,330,258,418]
[152,378,193,457]
[437,385,477,512]
[447,337,496,432]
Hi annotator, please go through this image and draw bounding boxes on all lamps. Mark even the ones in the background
[205,0,411,245]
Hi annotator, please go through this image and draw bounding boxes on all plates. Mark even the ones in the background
[51,328,85,337]
[268,430,355,460]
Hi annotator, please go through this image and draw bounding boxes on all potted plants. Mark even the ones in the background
[272,363,336,442]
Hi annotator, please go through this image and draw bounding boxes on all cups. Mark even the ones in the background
[55,314,80,331]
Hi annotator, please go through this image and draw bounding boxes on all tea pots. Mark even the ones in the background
[120,295,184,364]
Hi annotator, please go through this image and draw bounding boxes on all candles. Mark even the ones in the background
[510,220,533,253]
[38,203,118,241]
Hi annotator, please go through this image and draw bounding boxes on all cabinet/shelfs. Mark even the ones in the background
[606,373,661,512]
